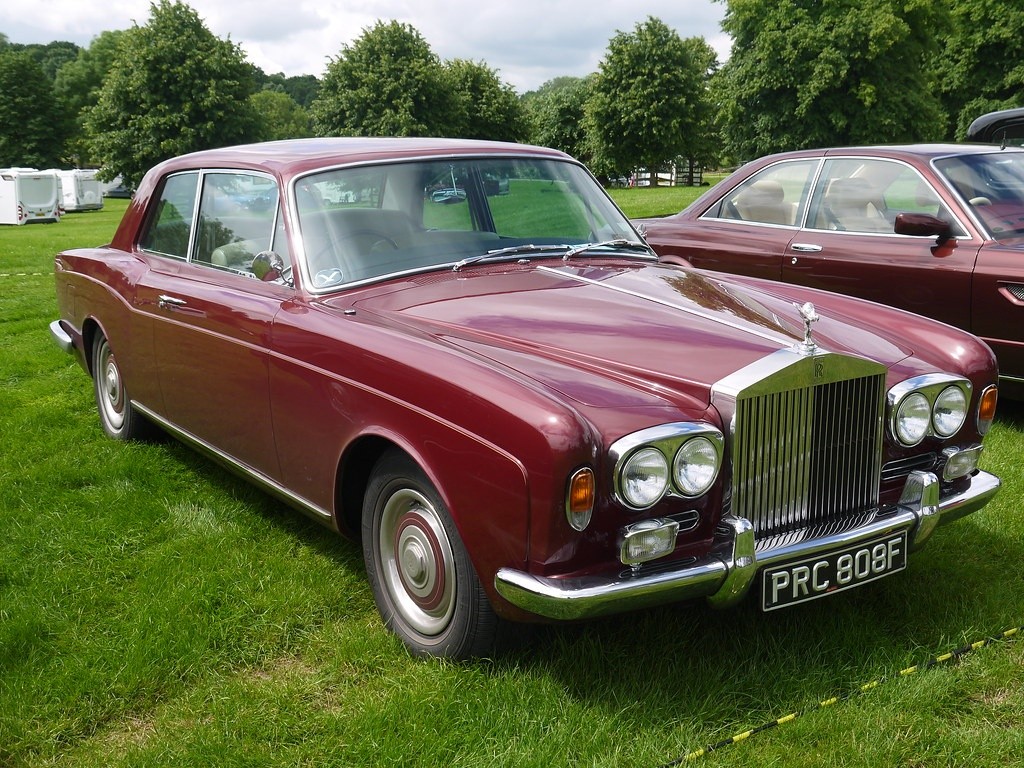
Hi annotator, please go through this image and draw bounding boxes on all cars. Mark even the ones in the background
[186,186,271,213]
[586,142,1024,381]
[102,184,137,200]
[44,133,1004,666]
[264,183,323,207]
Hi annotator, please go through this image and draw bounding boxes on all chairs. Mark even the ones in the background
[720,179,798,226]
[826,178,894,234]
[151,207,501,279]
